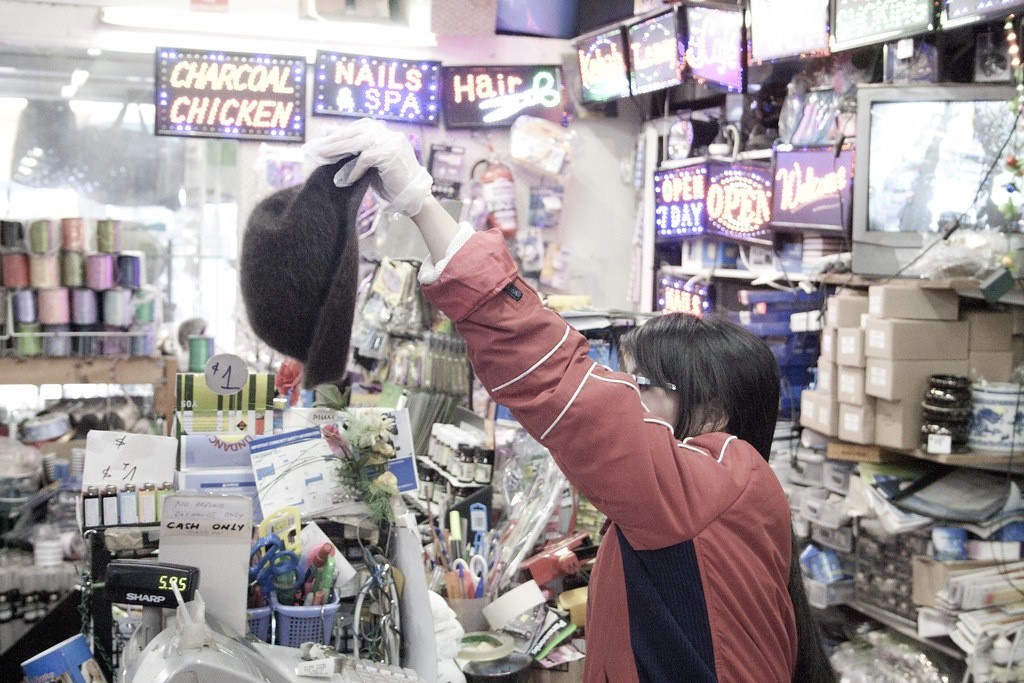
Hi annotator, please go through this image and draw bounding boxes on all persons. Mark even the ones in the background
[307,114,834,683]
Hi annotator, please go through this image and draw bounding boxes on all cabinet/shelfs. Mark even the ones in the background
[642,82,1024,683]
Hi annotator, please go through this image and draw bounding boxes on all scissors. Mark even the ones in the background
[248,535,298,589]
[452,555,489,598]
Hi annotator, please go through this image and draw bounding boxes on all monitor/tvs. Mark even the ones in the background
[851,82,1024,280]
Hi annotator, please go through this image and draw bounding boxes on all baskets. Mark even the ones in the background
[269,588,341,647]
[248,597,272,641]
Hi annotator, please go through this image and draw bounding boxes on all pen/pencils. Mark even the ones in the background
[459,566,466,599]
[291,541,340,607]
[434,511,518,593]
[254,587,264,608]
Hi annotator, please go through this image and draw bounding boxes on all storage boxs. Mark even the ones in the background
[800,284,1024,450]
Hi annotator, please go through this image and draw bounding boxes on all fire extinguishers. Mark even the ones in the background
[472,135,521,238]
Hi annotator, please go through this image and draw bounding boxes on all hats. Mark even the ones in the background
[240,155,380,389]
